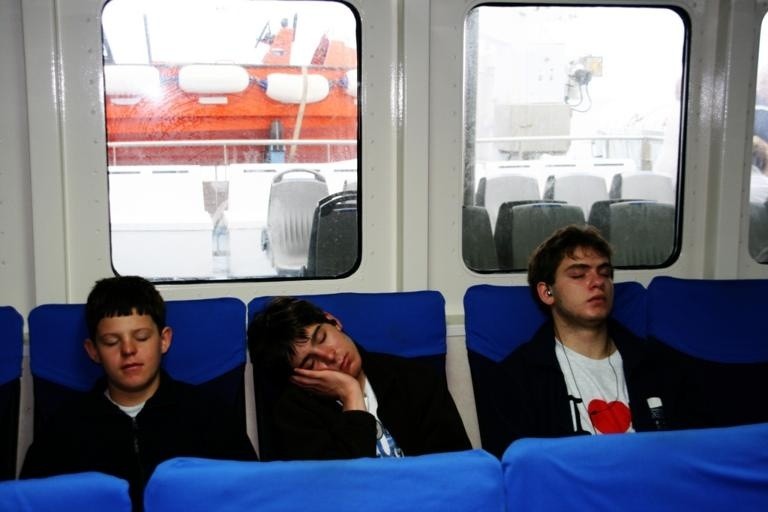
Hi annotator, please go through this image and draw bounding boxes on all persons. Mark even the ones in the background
[479,225,767,462]
[248,295,471,462]
[18,277,259,512]
[747,133,768,206]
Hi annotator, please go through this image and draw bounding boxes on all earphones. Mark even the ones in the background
[546,287,552,298]
